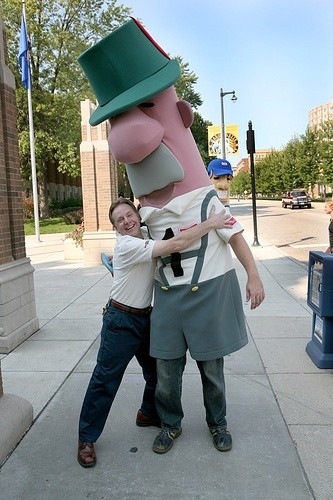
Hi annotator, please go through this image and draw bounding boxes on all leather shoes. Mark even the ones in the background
[77,436,97,468]
[137,409,166,428]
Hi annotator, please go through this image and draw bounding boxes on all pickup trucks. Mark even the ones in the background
[281,189,312,209]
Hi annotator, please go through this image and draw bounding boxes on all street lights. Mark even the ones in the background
[219,87,237,160]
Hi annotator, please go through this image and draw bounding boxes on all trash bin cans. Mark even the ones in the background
[304,249,333,371]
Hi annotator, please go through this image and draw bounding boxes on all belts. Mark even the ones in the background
[109,297,153,315]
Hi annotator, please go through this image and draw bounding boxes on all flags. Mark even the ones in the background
[17,15,33,91]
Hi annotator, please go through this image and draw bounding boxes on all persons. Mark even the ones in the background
[207,159,233,213]
[76,197,233,468]
[324,199,333,254]
[79,19,266,454]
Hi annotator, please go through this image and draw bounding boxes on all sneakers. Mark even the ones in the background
[208,424,233,451]
[152,421,182,453]
